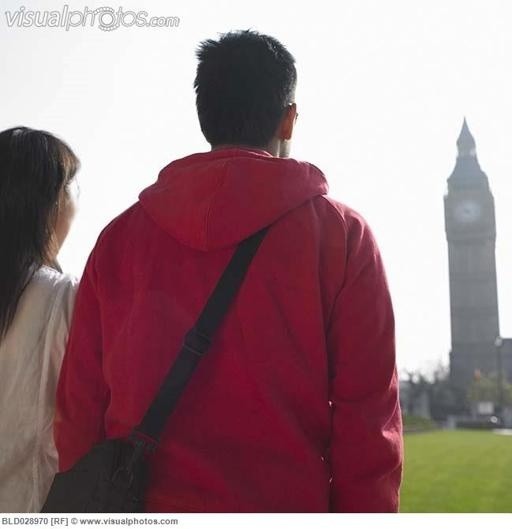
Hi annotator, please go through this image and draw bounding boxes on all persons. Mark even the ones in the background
[0,127,81,512]
[52,29,403,514]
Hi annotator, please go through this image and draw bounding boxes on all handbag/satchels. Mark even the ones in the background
[39,436,151,513]
